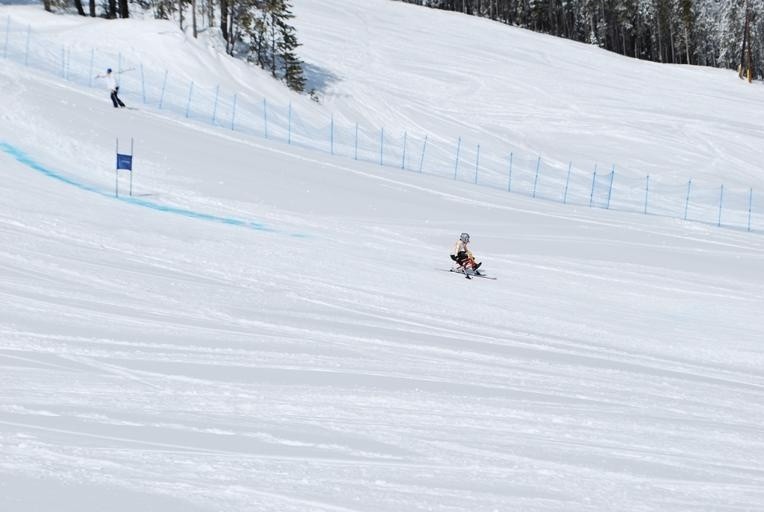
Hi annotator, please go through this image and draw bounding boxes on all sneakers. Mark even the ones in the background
[472,262,481,271]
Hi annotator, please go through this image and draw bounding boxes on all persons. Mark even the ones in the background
[449,232,483,271]
[103,68,125,109]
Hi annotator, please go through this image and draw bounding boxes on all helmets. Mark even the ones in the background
[460,233,470,243]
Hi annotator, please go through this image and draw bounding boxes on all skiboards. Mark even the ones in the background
[435,267,497,280]
